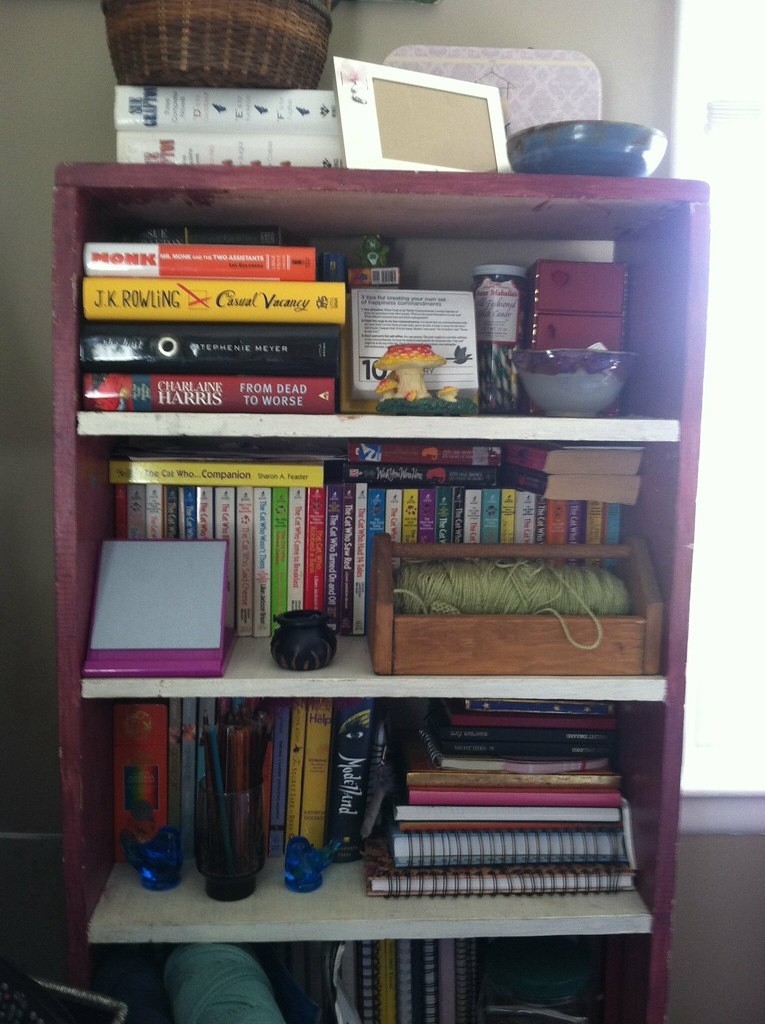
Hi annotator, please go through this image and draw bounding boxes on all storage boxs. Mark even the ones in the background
[113,85,347,168]
[370,531,663,678]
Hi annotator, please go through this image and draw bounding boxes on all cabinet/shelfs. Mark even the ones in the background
[54,162,710,1024]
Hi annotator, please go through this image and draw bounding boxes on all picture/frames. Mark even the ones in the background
[333,56,510,172]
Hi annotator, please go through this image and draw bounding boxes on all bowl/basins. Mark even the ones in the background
[505,120,668,180]
[513,348,641,418]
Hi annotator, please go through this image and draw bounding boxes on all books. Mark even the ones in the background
[114,697,638,899]
[282,939,491,1023]
[110,438,645,638]
[81,242,346,415]
[114,86,345,168]
